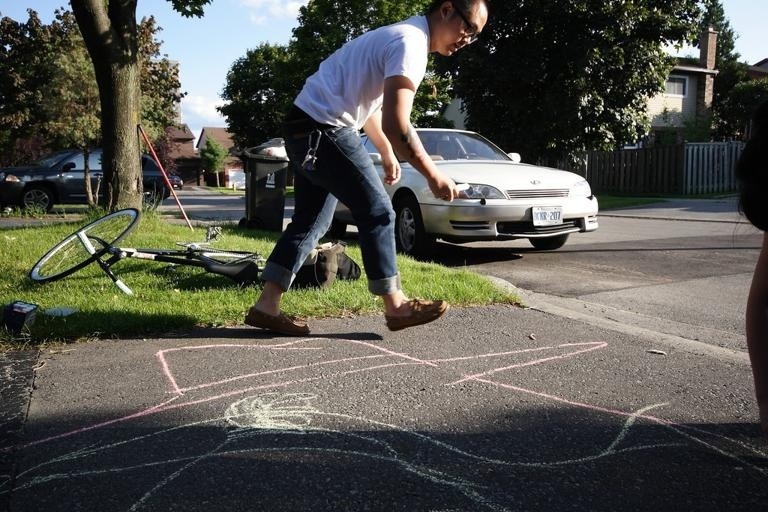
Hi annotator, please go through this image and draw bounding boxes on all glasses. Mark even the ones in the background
[452,2,481,43]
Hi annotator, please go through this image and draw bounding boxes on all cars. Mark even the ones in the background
[327,126,600,256]
[0,144,173,215]
[167,175,184,191]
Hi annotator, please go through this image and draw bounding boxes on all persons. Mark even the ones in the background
[733,101,768,443]
[243,0,488,334]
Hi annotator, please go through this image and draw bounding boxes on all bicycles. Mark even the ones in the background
[28,204,266,298]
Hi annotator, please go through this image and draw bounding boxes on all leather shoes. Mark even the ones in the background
[245,304,311,338]
[386,297,449,331]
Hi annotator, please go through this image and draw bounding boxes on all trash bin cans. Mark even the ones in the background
[237,137,290,233]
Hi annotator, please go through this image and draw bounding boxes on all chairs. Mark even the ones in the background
[432,135,457,160]
